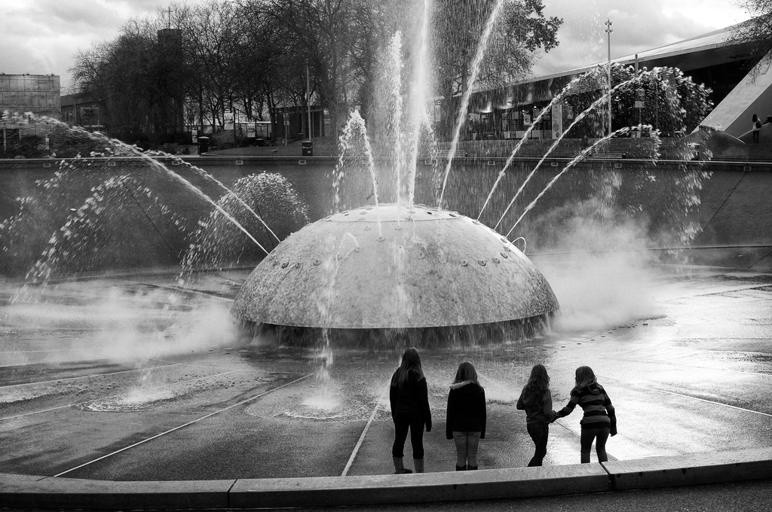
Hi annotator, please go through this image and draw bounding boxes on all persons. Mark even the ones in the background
[514,361,559,466]
[390,347,434,473]
[751,114,762,145]
[444,360,488,469]
[558,364,618,464]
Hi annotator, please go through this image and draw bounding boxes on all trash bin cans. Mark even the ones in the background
[302,140,313,157]
[197,136,210,155]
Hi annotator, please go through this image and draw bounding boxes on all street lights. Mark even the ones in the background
[604,18,613,139]
[305,55,312,141]
